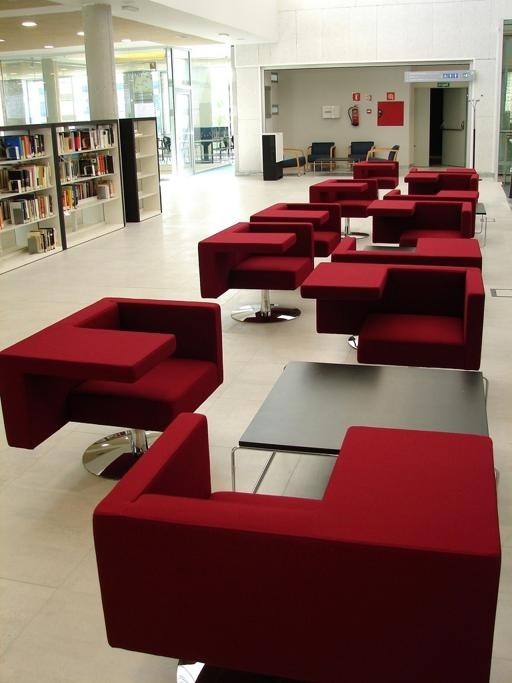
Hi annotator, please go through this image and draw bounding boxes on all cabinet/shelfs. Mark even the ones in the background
[122,116,163,223]
[53,119,127,250]
[1,123,66,283]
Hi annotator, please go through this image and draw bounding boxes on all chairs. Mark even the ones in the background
[403,166,479,194]
[0,294,225,482]
[197,220,316,326]
[330,234,487,271]
[382,188,480,212]
[352,160,400,189]
[298,261,487,372]
[279,139,401,177]
[308,178,379,240]
[249,202,342,258]
[93,410,504,681]
[157,127,234,165]
[365,199,475,247]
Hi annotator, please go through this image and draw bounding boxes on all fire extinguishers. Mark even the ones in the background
[348,106,359,126]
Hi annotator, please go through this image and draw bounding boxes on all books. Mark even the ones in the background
[0,120,116,254]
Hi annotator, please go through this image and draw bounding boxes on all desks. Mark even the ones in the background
[229,360,501,503]
